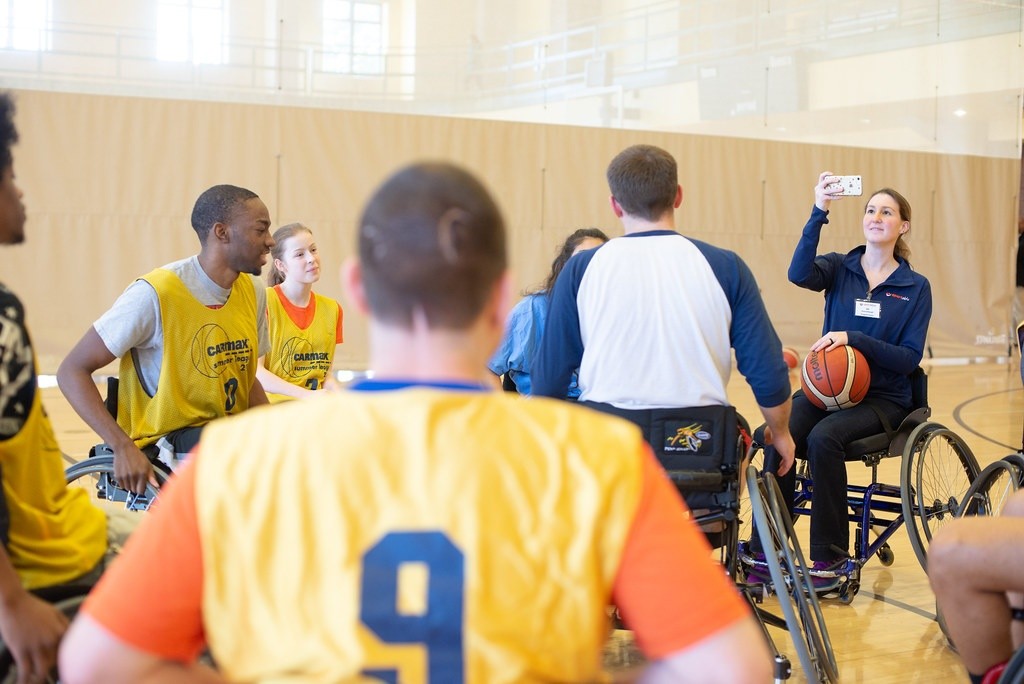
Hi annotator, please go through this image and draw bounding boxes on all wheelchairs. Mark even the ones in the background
[63,377,175,511]
[747,364,994,606]
[936,349,1024,650]
[574,398,841,684]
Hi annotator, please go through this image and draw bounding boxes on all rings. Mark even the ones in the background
[830,338,834,344]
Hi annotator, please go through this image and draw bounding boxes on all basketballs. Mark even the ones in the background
[782,348,799,369]
[800,345,870,411]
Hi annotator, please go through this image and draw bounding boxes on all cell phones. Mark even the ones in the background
[824,175,863,196]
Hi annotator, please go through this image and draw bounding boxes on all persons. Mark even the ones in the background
[60,161,775,684]
[256,223,344,403]
[56,184,276,494]
[926,488,1024,684]
[531,144,797,534]
[739,170,934,593]
[488,228,609,401]
[0,90,144,684]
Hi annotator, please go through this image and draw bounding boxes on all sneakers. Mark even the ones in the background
[802,552,850,591]
[745,552,786,597]
